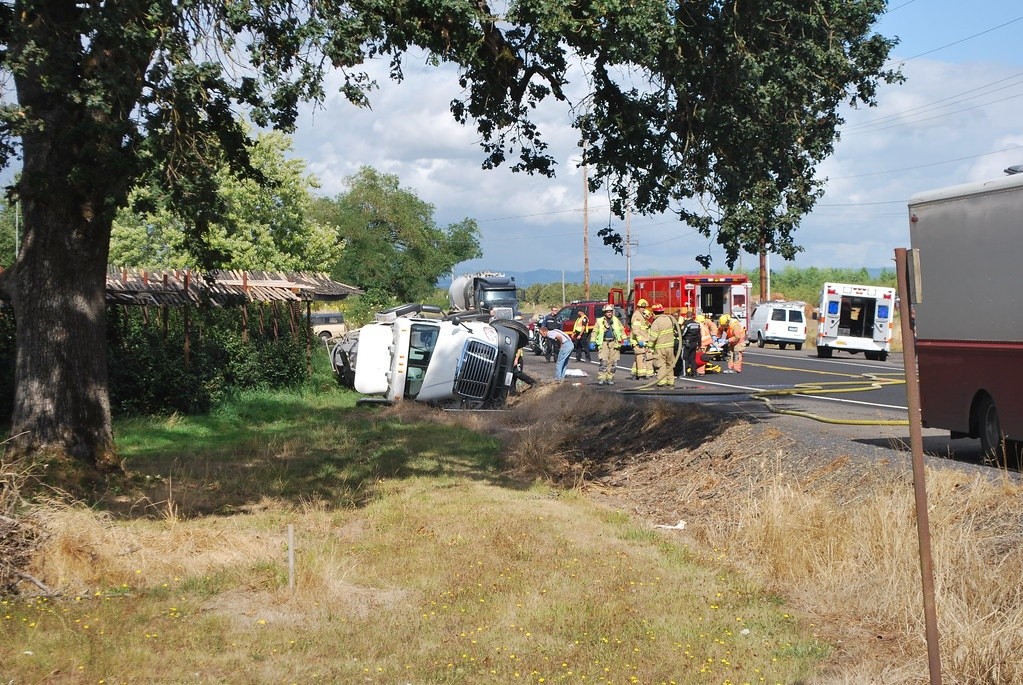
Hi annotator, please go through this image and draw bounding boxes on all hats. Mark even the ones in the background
[652,303,665,311]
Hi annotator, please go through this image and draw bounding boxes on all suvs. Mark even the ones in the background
[528,299,625,351]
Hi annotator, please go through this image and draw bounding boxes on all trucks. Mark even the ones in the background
[812,283,896,361]
[353,304,530,410]
[907,171,1023,467]
[448,272,525,315]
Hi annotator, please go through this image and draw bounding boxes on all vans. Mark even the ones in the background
[744,300,807,350]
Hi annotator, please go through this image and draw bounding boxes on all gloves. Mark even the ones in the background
[712,335,717,342]
[639,341,643,346]
[724,340,729,344]
[623,340,629,346]
[718,339,723,343]
[643,342,645,347]
[590,342,596,350]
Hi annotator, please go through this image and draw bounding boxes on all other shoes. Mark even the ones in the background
[598,381,604,385]
[657,384,664,390]
[667,384,674,390]
[573,360,581,363]
[723,368,737,374]
[585,362,591,365]
[608,380,614,385]
[624,375,653,382]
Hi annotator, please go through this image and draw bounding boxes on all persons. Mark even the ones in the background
[590,305,627,386]
[572,310,591,363]
[631,299,718,387]
[539,327,574,378]
[717,315,744,374]
[545,307,562,363]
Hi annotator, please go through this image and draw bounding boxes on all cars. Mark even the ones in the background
[302,313,345,340]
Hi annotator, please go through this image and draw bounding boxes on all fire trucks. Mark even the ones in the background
[609,274,751,339]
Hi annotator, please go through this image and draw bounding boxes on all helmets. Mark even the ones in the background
[637,299,649,306]
[642,309,651,319]
[720,313,731,325]
[695,315,706,323]
[601,304,613,312]
